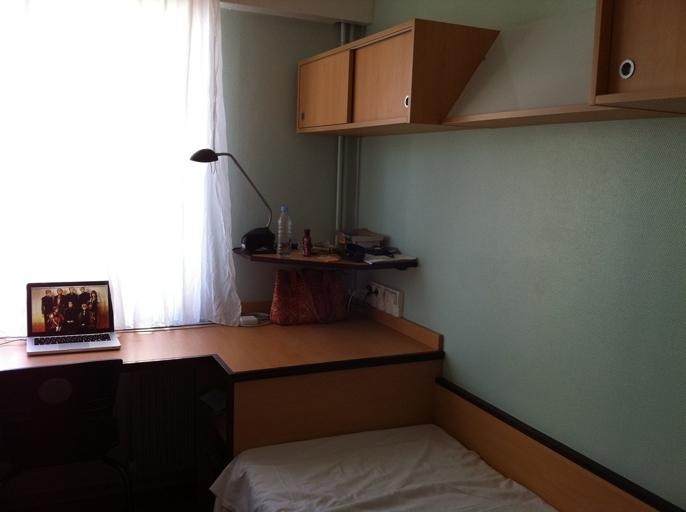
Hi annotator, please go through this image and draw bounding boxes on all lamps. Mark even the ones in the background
[190,149,276,254]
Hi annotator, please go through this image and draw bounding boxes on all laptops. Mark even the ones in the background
[25,280,121,355]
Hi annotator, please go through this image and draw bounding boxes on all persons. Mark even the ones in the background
[42,287,100,332]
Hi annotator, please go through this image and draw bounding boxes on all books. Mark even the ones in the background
[336,228,419,265]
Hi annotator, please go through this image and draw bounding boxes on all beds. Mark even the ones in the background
[209,423,563,511]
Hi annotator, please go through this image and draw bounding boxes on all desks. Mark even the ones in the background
[1,304,446,458]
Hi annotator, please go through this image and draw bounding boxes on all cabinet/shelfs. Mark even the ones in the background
[296,17,501,135]
[588,0,685,122]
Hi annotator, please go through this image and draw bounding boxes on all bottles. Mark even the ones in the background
[303,229,312,257]
[276,207,294,255]
